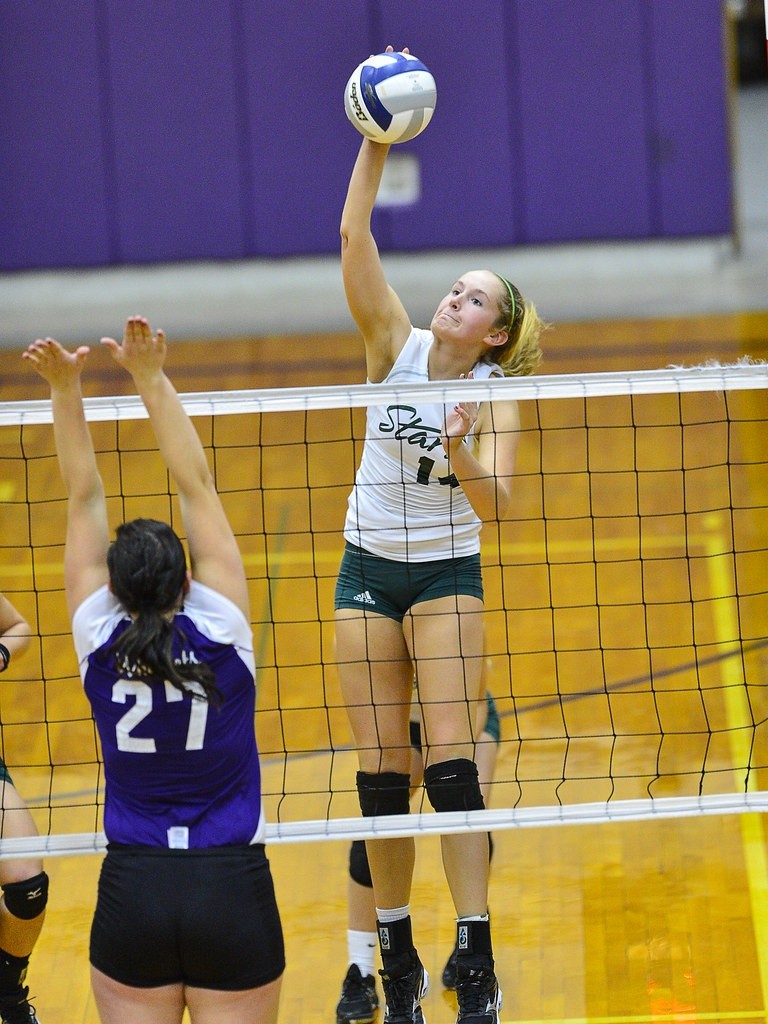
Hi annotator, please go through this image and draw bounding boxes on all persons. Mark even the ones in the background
[331,46,539,1024]
[0,576,51,1021]
[18,315,289,1024]
[334,600,505,1024]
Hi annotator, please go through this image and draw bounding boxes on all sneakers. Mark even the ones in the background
[336,964,380,1024]
[379,957,429,1024]
[1,986,39,1024]
[442,946,461,989]
[453,964,502,1024]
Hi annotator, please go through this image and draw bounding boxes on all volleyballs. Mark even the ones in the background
[345,52,436,146]
